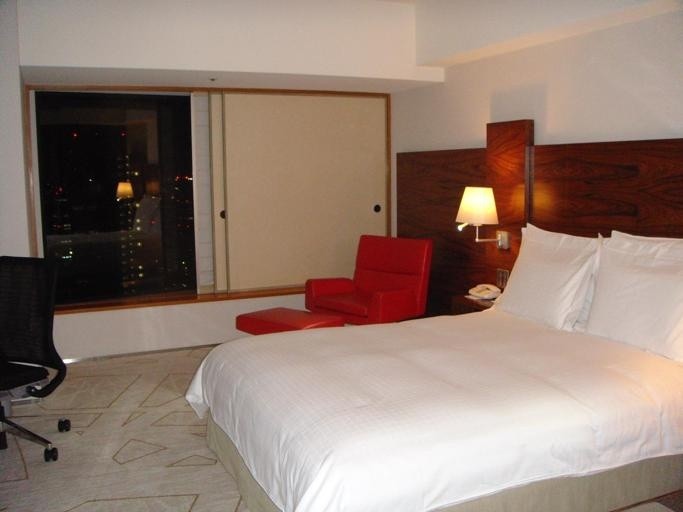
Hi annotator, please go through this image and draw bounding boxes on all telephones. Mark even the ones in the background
[468,284,501,299]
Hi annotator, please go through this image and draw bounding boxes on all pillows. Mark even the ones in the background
[491,223,681,362]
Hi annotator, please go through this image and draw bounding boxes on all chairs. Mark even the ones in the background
[1,254,72,463]
[304,234,434,326]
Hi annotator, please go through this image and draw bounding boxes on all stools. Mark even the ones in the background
[234,307,347,335]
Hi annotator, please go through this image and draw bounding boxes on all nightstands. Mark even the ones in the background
[448,286,500,315]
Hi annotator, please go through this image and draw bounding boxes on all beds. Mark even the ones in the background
[201,221,683,511]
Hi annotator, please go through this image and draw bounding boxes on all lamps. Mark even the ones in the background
[114,179,135,201]
[453,186,508,253]
